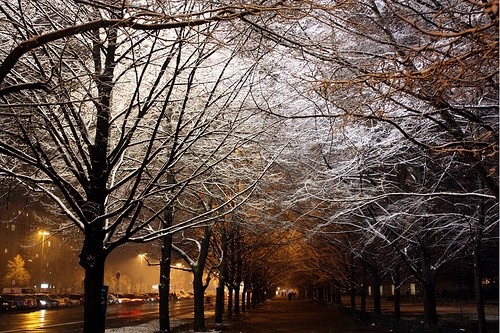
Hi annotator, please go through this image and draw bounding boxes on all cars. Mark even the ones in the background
[0,286,229,313]
